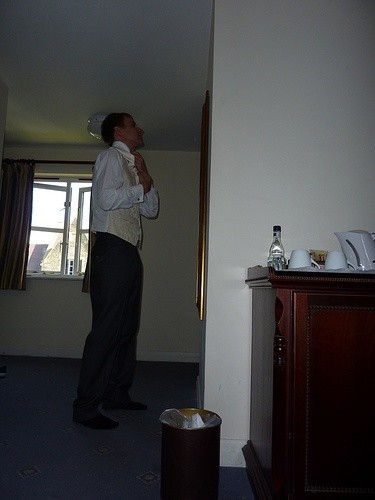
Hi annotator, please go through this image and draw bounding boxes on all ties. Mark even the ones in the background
[133,167,139,185]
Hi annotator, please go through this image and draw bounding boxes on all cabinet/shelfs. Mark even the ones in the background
[241,267,375,500]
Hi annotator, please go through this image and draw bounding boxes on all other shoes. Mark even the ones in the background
[0,365,8,377]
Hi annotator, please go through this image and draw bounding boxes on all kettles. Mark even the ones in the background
[333,230,375,271]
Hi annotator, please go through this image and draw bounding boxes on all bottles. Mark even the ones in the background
[269,226,285,269]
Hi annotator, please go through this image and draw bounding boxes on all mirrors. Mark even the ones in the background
[196,90,209,321]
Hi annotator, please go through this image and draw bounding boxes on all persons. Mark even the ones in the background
[73,113,158,430]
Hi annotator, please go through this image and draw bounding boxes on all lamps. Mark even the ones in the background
[87,117,105,140]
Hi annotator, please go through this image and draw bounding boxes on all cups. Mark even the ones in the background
[288,250,320,269]
[324,252,356,270]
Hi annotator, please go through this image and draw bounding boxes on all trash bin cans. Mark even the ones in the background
[159,406,223,500]
